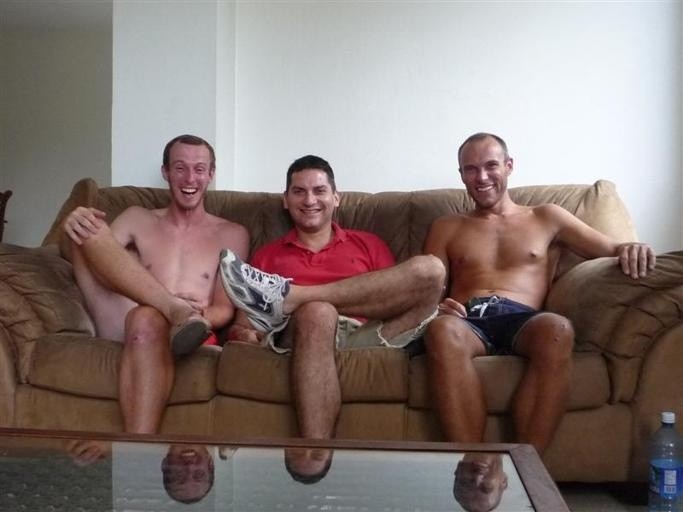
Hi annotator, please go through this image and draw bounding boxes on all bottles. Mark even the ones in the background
[649,412,683,512]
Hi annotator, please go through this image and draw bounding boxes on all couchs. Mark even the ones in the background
[0,177,683,484]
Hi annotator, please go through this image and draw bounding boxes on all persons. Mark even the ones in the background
[57,132,251,435]
[60,438,216,505]
[217,155,449,440]
[218,444,335,486]
[423,131,658,461]
[452,453,508,512]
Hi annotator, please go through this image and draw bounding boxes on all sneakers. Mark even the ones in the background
[166,316,213,355]
[217,249,293,334]
